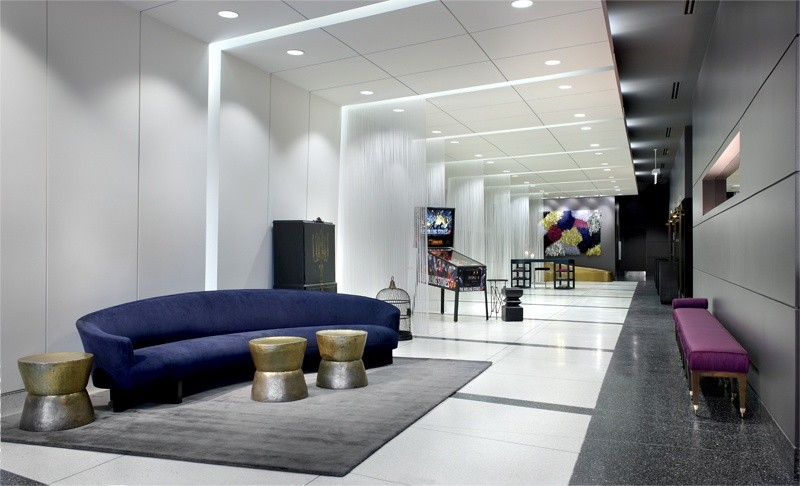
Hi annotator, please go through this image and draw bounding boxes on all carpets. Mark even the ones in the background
[0,357,492,477]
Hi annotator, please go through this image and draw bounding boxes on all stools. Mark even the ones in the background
[486,278,508,319]
[534,267,551,290]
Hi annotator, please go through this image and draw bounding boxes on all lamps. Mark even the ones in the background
[651,148,661,184]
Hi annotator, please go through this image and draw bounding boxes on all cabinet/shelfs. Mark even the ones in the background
[272,218,337,293]
[510,258,575,290]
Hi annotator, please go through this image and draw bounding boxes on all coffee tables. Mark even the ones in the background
[19,353,93,432]
[315,329,369,391]
[249,336,307,403]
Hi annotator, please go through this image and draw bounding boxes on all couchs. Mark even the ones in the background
[75,288,401,413]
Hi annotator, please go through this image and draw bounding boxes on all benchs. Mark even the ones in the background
[672,298,751,418]
